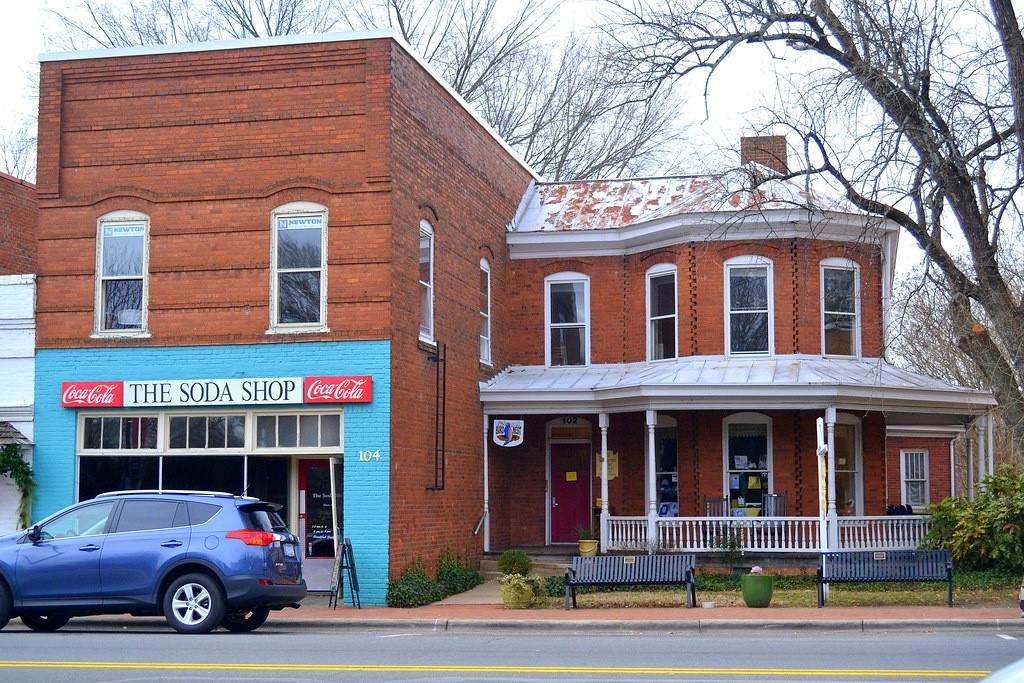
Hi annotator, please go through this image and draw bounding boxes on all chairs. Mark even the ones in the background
[700,495,729,547]
[751,492,789,548]
[888,504,921,542]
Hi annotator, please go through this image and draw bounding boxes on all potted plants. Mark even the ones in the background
[496,549,535,609]
[574,522,600,556]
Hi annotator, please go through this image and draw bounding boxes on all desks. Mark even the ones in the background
[731,507,761,517]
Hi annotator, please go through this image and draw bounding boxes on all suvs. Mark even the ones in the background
[1,489,308,634]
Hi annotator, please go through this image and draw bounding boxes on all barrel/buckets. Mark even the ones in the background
[578,540,598,556]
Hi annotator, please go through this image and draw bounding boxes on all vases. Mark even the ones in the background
[741,574,774,608]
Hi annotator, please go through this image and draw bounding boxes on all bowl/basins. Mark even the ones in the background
[701,601,715,608]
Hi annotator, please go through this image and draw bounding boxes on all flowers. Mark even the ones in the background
[749,566,765,576]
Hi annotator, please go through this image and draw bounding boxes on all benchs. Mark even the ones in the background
[564,554,697,610]
[817,549,953,610]
